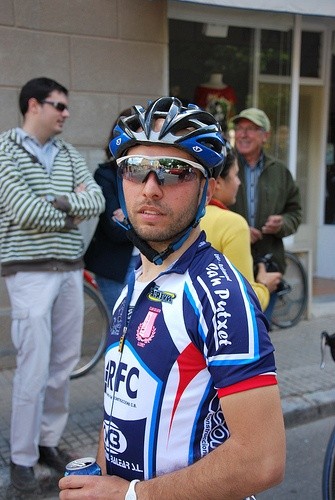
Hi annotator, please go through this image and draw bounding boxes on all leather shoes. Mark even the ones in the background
[38,445,75,473]
[10,459,36,492]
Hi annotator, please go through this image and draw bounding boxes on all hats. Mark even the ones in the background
[231,108,271,133]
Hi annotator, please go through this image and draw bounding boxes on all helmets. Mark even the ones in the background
[109,97,228,180]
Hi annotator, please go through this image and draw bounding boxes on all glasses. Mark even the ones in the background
[38,101,68,111]
[116,155,208,186]
[234,126,261,133]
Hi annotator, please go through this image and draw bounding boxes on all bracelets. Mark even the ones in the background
[125,479,141,500]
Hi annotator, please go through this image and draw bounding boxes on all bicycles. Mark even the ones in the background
[72,265,109,379]
[271,251,308,329]
[318,330,335,499]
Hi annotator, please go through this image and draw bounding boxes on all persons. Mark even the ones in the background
[0,78,105,490]
[58,96,289,500]
[85,105,305,330]
[195,73,237,132]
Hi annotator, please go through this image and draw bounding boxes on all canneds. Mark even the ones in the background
[63,457,103,476]
